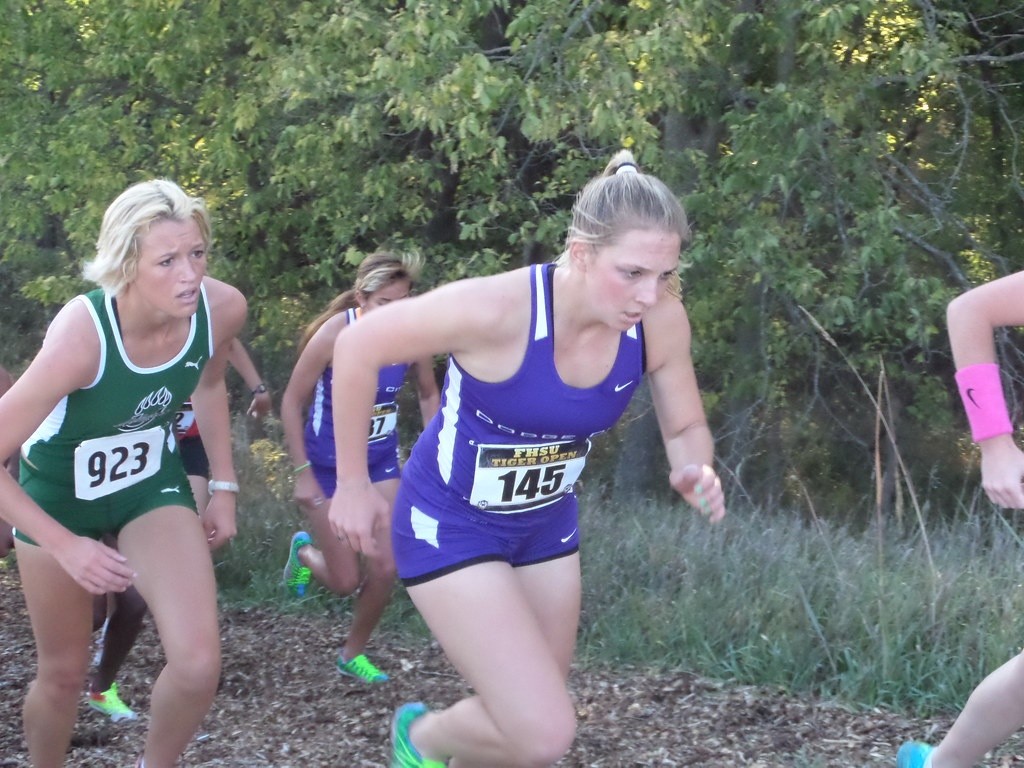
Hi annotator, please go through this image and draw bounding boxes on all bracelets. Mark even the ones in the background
[207,479,240,496]
[294,464,310,472]
[252,383,267,395]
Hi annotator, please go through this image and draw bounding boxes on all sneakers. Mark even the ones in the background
[391,702,446,768]
[284,531,311,597]
[336,654,390,684]
[896,740,932,768]
[90,682,137,722]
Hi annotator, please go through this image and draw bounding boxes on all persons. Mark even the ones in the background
[328,149,724,768]
[896,271,1024,768]
[89,336,272,721]
[0,178,247,768]
[278,254,439,685]
